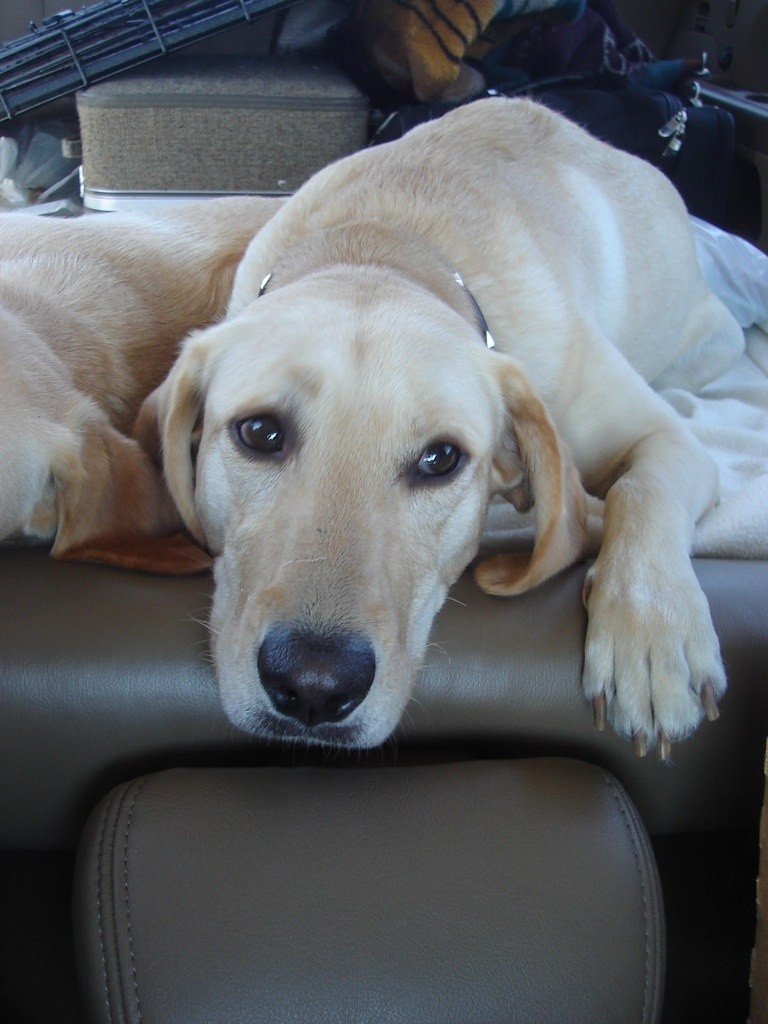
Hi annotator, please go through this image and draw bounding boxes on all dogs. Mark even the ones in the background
[1,96,744,759]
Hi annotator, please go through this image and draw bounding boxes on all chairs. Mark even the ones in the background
[0,554,766,1024]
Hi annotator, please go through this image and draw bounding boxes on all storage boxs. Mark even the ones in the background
[78,54,371,214]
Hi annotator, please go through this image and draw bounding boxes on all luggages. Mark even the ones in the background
[640,75,736,229]
[63,54,369,216]
